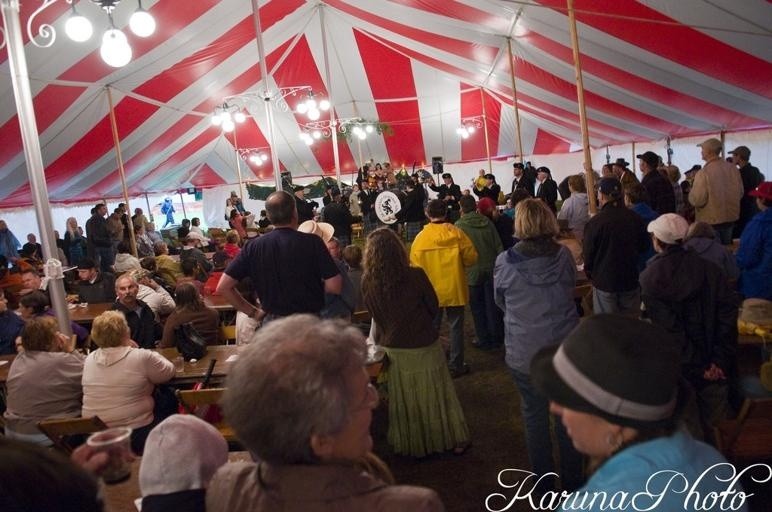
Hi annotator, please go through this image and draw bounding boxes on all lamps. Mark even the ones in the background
[296,91,330,121]
[454,118,483,139]
[250,151,268,167]
[62,0,156,68]
[212,101,245,133]
[300,126,330,145]
[348,119,375,141]
[215,325,236,345]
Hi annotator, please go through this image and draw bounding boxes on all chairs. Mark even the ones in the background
[173,387,243,444]
[33,414,107,460]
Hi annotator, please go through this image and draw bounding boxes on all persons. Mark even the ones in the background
[140,257,175,299]
[687,138,737,246]
[53,232,67,264]
[146,224,163,246]
[409,199,472,377]
[684,220,747,415]
[583,177,647,318]
[162,197,176,228]
[523,162,537,196]
[229,209,248,240]
[357,179,378,231]
[4,318,88,461]
[297,220,357,327]
[505,197,512,208]
[373,164,385,191]
[89,272,154,354]
[0,288,24,434]
[78,228,93,263]
[18,290,89,349]
[426,173,461,224]
[225,192,240,229]
[105,207,125,265]
[472,174,500,206]
[529,313,751,511]
[293,185,316,228]
[203,252,229,298]
[0,221,23,259]
[216,192,342,325]
[414,174,426,220]
[133,209,146,235]
[685,165,701,187]
[83,310,176,459]
[495,199,587,509]
[222,229,244,263]
[680,181,694,225]
[726,156,734,164]
[342,245,373,333]
[119,203,132,253]
[606,159,637,191]
[666,165,685,220]
[637,152,675,216]
[0,254,32,303]
[66,257,114,305]
[324,187,365,250]
[558,176,572,200]
[177,220,204,254]
[0,414,230,512]
[556,176,591,246]
[181,231,213,283]
[382,162,396,187]
[478,198,517,340]
[732,182,772,320]
[25,234,44,264]
[65,217,84,267]
[159,283,216,350]
[255,211,270,235]
[503,190,526,219]
[454,195,504,351]
[603,164,614,177]
[557,220,589,324]
[153,242,186,295]
[479,170,485,201]
[326,236,341,258]
[16,270,52,315]
[87,205,114,271]
[206,313,447,512]
[536,167,558,219]
[362,228,471,458]
[623,184,660,273]
[175,255,204,296]
[112,253,176,340]
[321,195,332,222]
[636,213,738,454]
[190,219,217,252]
[134,225,155,259]
[348,185,363,216]
[729,150,765,244]
[236,198,251,216]
[395,181,422,243]
[505,163,531,206]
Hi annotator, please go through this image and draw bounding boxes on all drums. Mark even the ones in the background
[375,188,407,225]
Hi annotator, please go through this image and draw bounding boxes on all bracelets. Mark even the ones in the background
[15,343,24,350]
[70,349,76,354]
[248,306,256,318]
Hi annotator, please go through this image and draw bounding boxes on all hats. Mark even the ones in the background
[536,167,551,174]
[636,151,659,167]
[597,176,623,197]
[696,138,723,153]
[77,259,95,269]
[748,181,772,200]
[483,174,494,180]
[727,146,752,158]
[514,163,524,169]
[529,311,689,429]
[478,196,496,212]
[611,158,630,167]
[646,211,691,245]
[296,219,336,243]
[136,413,229,512]
[187,231,200,240]
[442,173,452,179]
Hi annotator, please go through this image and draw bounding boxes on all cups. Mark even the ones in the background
[86,426,133,486]
[733,238,740,249]
[173,356,184,373]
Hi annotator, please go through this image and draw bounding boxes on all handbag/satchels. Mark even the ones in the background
[173,322,208,361]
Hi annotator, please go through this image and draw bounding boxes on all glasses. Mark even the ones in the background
[349,383,379,415]
